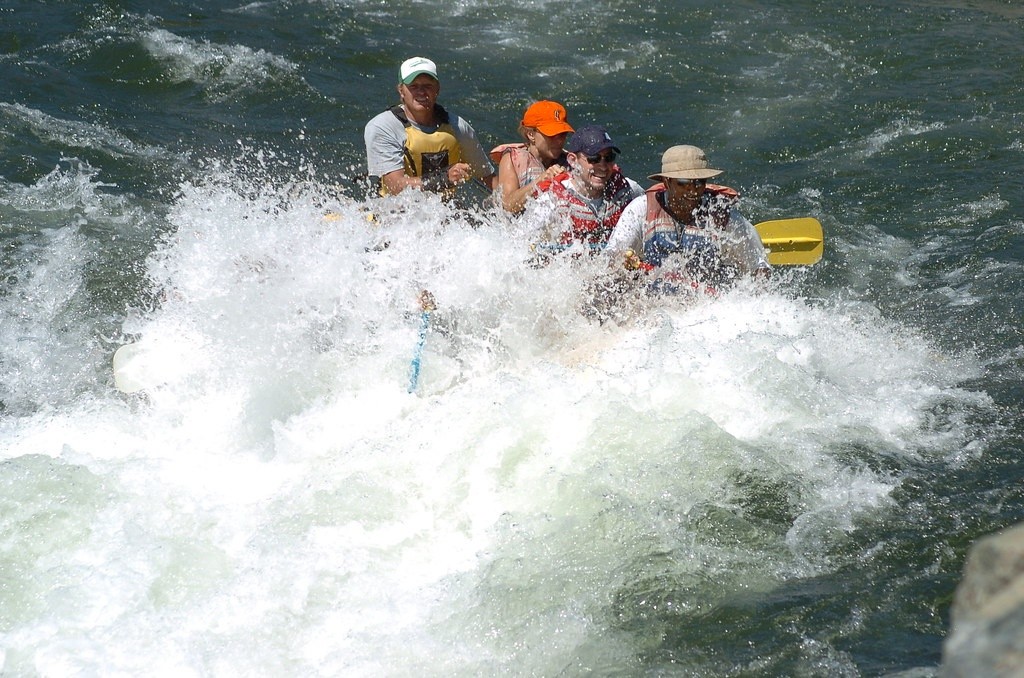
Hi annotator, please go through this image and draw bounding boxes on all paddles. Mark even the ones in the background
[753,217,824,265]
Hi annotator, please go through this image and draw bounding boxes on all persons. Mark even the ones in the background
[490,101,576,213]
[533,125,645,244]
[364,57,499,196]
[599,145,770,276]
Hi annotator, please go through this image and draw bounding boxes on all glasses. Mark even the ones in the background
[577,151,617,164]
[669,179,707,188]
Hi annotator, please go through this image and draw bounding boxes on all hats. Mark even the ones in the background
[398,57,439,84]
[521,99,575,136]
[569,126,621,156]
[647,145,724,181]
